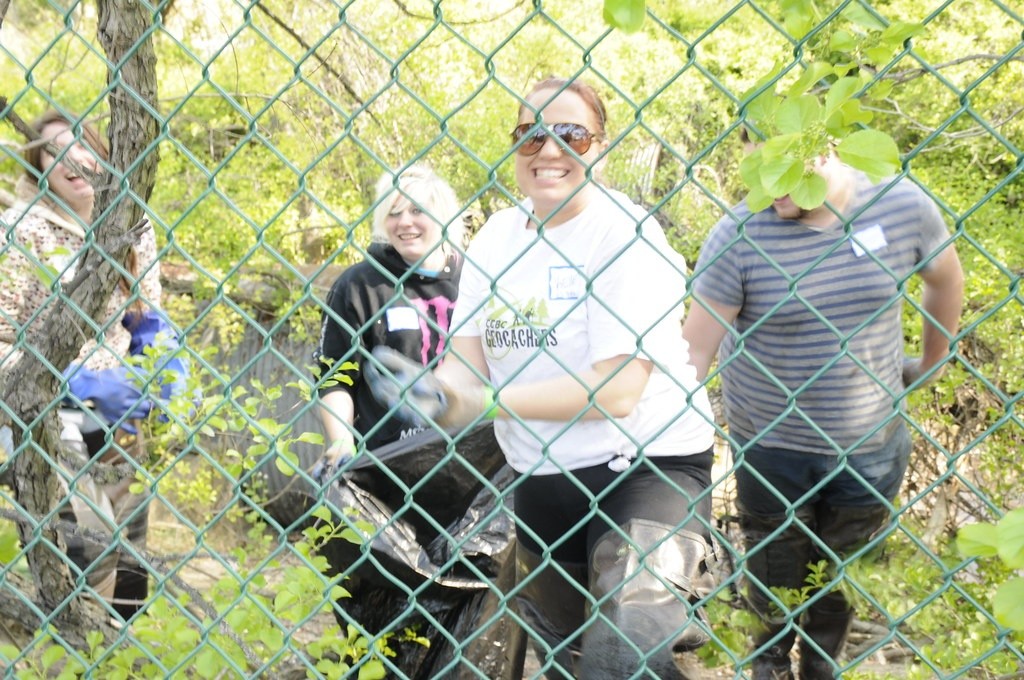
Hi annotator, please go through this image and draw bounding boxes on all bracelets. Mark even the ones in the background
[482,385,500,421]
[332,438,358,458]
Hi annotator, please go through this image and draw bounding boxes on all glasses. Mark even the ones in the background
[511,124,600,156]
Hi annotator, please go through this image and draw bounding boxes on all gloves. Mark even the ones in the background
[122,312,203,424]
[57,364,154,435]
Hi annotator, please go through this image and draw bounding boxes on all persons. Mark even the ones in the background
[366,77,719,679]
[682,91,967,680]
[315,164,506,680]
[0,110,164,632]
